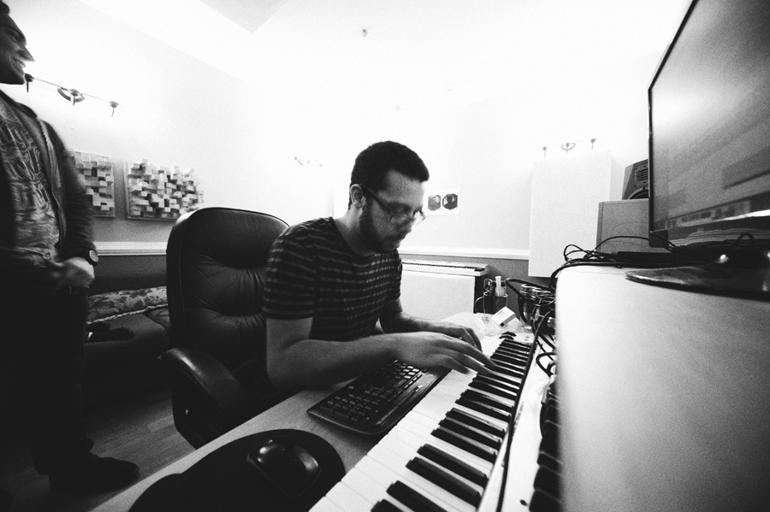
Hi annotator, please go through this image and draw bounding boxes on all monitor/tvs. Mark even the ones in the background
[625,0,769,301]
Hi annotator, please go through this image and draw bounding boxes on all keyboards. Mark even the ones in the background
[307,360,453,442]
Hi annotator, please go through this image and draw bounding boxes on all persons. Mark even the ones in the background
[0,3,139,502]
[260,141,494,397]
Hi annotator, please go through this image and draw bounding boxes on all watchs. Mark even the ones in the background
[73,244,99,267]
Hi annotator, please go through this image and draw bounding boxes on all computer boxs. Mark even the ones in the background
[596,200,671,256]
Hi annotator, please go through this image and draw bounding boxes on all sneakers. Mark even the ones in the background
[49,454,139,491]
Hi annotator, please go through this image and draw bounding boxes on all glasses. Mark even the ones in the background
[361,184,425,224]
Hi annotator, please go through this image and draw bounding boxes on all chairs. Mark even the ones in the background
[152,207,292,451]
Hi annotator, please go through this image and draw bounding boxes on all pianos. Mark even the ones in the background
[308,330,546,512]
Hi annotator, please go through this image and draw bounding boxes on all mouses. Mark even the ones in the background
[247,440,322,498]
